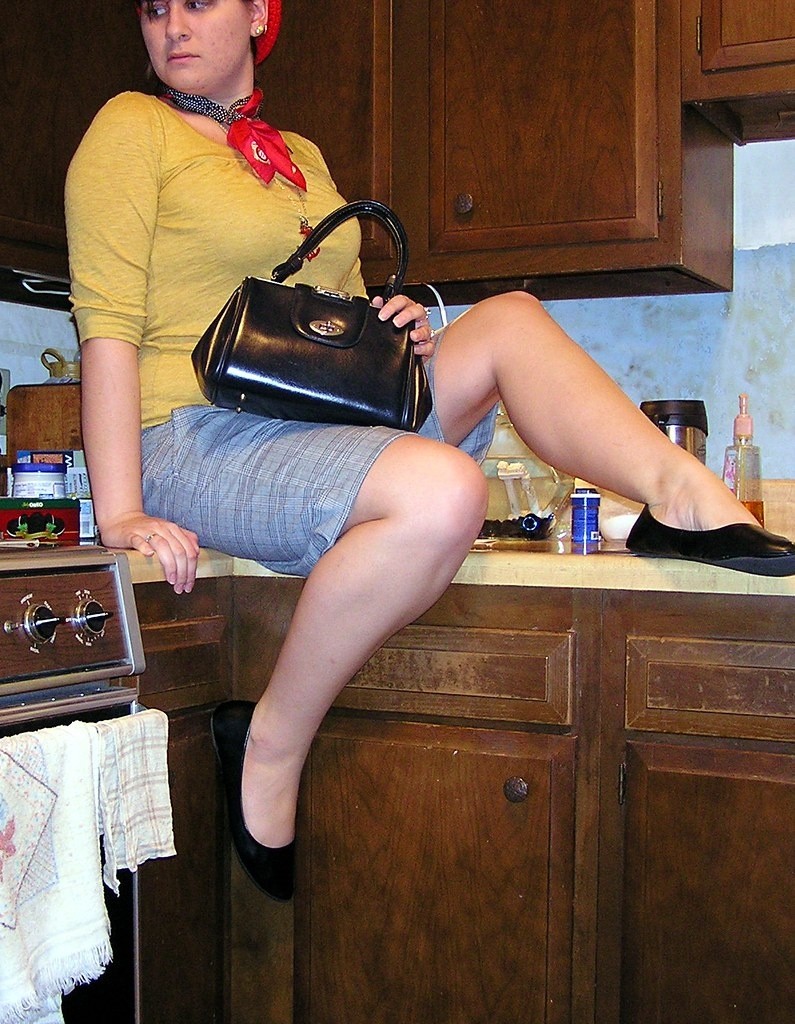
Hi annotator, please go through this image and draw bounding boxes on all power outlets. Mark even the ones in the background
[0,368,11,435]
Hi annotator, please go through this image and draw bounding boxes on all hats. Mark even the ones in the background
[137,0,282,65]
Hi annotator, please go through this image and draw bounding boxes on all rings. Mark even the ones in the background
[419,307,431,321]
[431,329,434,338]
[146,533,158,543]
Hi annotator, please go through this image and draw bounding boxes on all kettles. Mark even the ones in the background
[640,400,708,465]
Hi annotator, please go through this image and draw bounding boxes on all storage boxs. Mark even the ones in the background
[79,499,96,538]
[0,498,81,542]
[16,449,86,468]
[7,467,92,499]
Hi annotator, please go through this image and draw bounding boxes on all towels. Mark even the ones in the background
[0,707,175,1024]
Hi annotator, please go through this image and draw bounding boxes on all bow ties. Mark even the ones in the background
[162,89,307,192]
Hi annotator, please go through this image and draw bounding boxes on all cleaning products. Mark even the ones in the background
[721,392,767,535]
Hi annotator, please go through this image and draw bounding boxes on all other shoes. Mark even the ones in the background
[210,699,296,904]
[624,503,795,577]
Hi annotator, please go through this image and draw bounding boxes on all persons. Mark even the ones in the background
[62,2,793,903]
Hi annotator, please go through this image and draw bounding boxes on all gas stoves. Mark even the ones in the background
[0,552,146,696]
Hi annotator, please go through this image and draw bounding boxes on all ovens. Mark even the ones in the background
[0,687,139,1024]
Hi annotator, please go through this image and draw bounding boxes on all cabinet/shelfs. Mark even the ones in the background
[617,635,795,1024]
[428,0,662,254]
[0,0,160,253]
[291,624,598,1024]
[256,0,393,263]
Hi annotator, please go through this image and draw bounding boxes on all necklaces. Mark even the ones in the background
[208,115,315,237]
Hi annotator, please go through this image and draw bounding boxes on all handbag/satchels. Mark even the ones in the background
[191,200,433,433]
